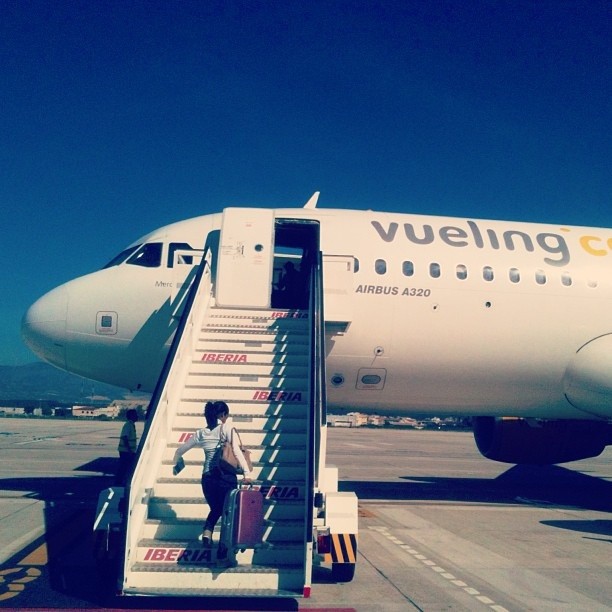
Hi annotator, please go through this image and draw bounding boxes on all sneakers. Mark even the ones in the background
[203,530,213,548]
[216,559,233,567]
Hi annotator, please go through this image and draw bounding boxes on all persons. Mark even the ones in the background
[277,261,299,291]
[118,408,139,469]
[174,400,254,569]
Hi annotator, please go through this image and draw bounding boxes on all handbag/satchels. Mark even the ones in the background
[209,424,237,487]
[220,427,253,474]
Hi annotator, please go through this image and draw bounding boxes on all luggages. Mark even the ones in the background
[220,480,264,553]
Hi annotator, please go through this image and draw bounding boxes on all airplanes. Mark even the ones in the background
[21,191,612,597]
